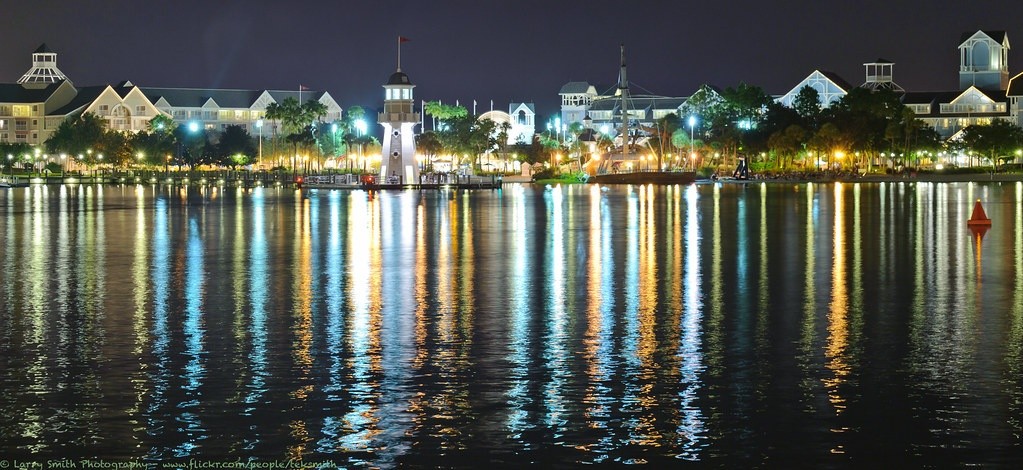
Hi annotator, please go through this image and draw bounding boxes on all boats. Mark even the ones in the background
[695,179,718,183]
[0,181,12,188]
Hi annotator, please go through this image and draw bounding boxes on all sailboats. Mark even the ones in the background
[589,44,697,184]
